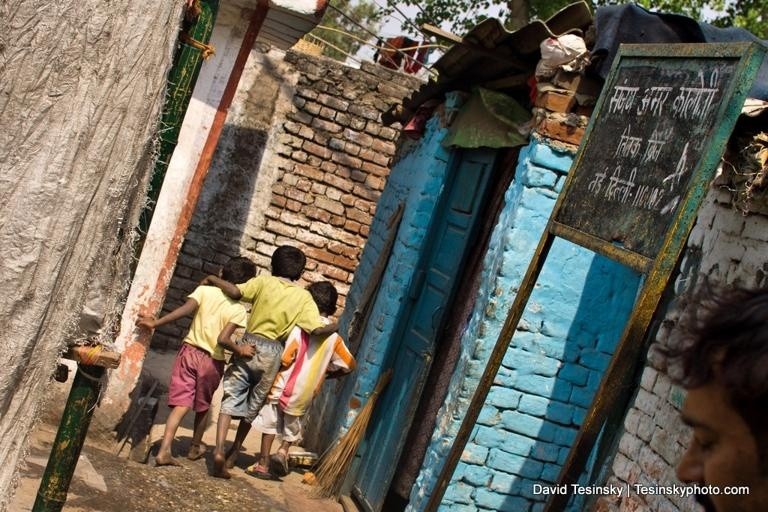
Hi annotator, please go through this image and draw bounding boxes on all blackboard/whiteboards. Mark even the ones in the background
[549,41,768,273]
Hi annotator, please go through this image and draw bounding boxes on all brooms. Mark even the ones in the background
[305,368,395,506]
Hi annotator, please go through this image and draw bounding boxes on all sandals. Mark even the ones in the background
[245,448,289,478]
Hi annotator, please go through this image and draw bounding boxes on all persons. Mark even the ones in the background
[677,282,766,511]
[200,246,340,477]
[135,255,255,465]
[245,279,356,477]
